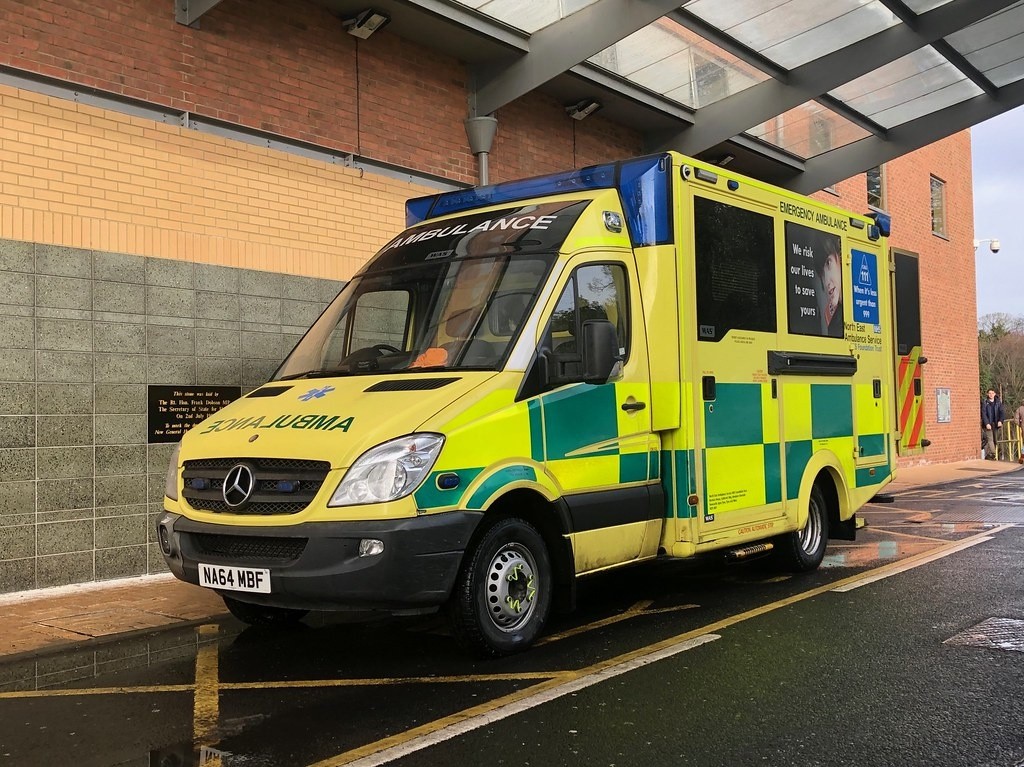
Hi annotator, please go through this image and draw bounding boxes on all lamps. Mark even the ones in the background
[566,98,603,122]
[340,4,389,39]
[709,152,737,170]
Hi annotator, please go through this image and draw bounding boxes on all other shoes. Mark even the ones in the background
[1019,454,1024,464]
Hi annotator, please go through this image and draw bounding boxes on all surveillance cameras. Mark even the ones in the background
[989,242,1000,253]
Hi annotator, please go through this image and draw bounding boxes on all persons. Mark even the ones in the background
[981,387,1004,460]
[1013,401,1024,464]
[813,232,844,337]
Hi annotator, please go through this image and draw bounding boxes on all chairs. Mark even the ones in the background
[437,309,496,369]
[552,306,614,356]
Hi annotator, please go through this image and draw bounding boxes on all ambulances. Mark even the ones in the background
[152,148,932,658]
